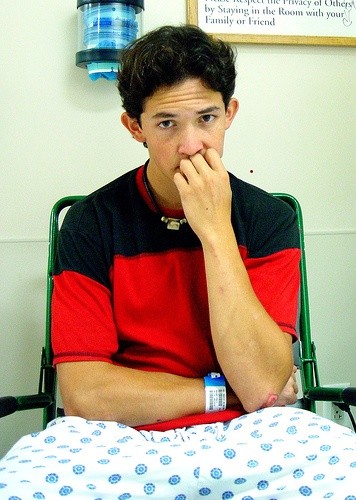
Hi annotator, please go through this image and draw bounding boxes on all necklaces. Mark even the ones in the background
[142,158,188,230]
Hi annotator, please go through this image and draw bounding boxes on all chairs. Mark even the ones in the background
[0,193,356,433]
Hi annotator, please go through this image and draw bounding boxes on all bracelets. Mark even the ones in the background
[203,371,228,412]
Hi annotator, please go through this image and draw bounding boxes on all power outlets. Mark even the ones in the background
[323,383,349,428]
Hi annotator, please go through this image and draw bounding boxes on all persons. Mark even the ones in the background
[50,25,303,431]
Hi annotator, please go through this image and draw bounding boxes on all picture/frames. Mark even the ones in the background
[185,0,356,47]
